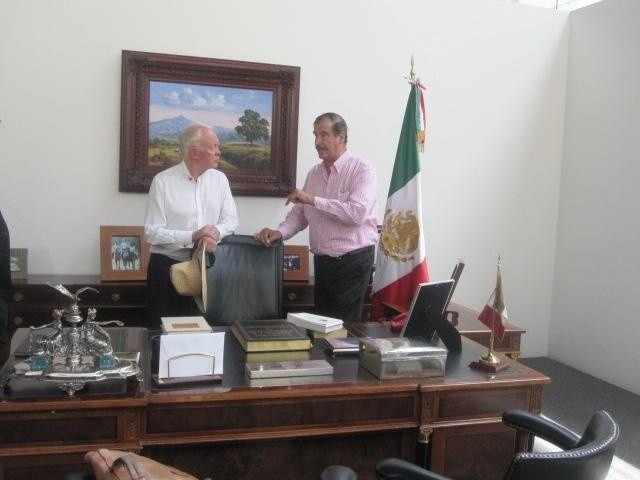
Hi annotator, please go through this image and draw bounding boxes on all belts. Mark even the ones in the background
[322,244,374,261]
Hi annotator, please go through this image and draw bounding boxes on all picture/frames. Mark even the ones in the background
[283,246,309,281]
[432,263,465,344]
[119,50,300,198]
[100,225,149,282]
[399,280,462,352]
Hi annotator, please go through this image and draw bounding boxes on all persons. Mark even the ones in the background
[252,112,380,322]
[112,242,138,270]
[144,124,239,327]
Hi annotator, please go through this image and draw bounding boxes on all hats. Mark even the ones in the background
[170,240,208,314]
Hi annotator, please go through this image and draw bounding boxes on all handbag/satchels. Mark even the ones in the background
[84,447,199,480]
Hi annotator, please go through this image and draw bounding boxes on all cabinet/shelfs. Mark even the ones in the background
[0,274,371,328]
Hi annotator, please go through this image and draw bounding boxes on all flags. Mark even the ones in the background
[369,78,433,313]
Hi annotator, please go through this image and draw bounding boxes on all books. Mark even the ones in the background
[159,315,213,334]
[231,319,312,352]
[286,311,343,334]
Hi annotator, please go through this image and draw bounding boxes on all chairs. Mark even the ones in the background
[373,409,619,480]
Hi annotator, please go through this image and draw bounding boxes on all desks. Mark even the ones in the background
[0,321,551,480]
[446,301,526,361]
